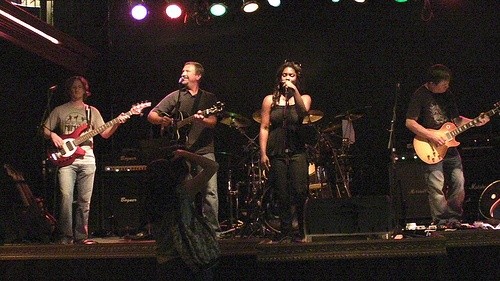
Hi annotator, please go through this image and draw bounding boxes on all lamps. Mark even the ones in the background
[241,0,260,14]
[129,0,150,21]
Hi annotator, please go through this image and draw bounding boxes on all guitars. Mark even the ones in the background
[413,101,500,165]
[3,163,59,239]
[46,99,152,168]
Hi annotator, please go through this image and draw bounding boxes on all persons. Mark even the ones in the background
[41,76,130,244]
[148,62,220,237]
[406,64,490,228]
[259,62,311,241]
[147,150,219,281]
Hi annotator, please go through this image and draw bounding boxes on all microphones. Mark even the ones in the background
[47,83,61,91]
[178,77,184,83]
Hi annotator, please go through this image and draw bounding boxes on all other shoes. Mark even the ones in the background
[434,220,447,229]
[448,222,467,229]
[59,236,74,244]
[74,237,97,244]
[295,236,307,242]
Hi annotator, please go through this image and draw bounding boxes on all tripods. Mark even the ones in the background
[218,131,278,236]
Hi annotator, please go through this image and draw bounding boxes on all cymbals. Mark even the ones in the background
[252,108,262,124]
[220,111,253,128]
[319,123,342,133]
[302,109,324,124]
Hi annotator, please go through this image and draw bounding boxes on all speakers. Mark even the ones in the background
[459,155,500,223]
[51,169,103,232]
[388,161,432,220]
[301,195,392,238]
[102,171,149,235]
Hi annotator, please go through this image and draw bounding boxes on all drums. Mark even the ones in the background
[246,174,266,205]
[219,166,251,184]
[215,151,234,181]
[307,158,327,192]
[259,186,299,234]
[242,145,260,164]
